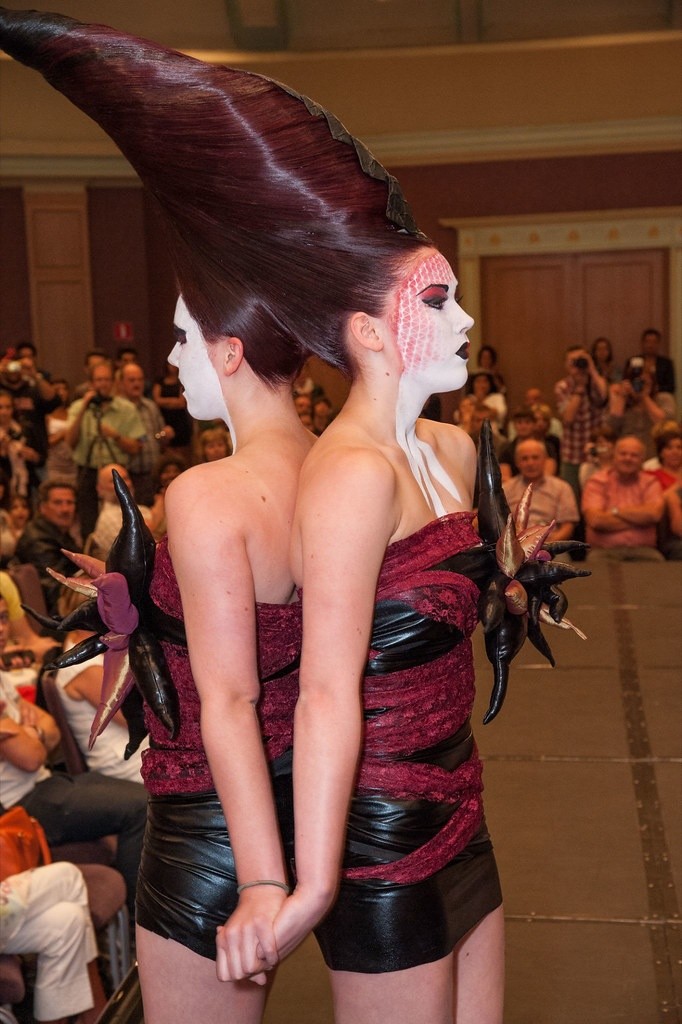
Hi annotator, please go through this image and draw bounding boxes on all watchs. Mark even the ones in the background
[611,507,619,514]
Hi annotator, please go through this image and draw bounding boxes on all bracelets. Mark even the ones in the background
[237,880,289,896]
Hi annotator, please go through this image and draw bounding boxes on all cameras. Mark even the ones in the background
[631,378,645,392]
[8,361,22,372]
[572,357,589,367]
[88,394,113,411]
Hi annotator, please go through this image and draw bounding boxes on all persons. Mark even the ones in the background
[0,0,682,1024]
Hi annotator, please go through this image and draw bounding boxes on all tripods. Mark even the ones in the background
[76,412,118,507]
